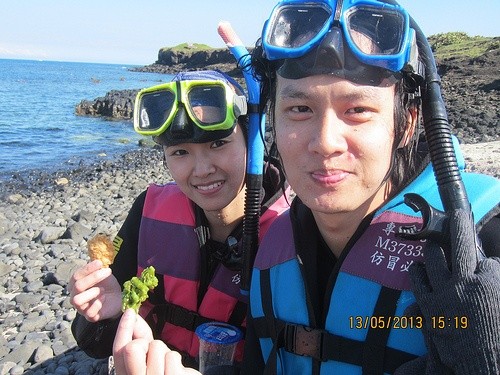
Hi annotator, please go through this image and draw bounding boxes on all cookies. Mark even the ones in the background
[87,233,115,268]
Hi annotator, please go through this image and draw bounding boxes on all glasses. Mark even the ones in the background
[132,79,238,135]
[262,1,420,72]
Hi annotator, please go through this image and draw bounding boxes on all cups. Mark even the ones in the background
[195,321,242,375]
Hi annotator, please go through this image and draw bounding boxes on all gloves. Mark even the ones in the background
[406,211,500,374]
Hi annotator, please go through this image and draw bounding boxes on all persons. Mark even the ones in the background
[112,0,500,375]
[68,69,296,375]
